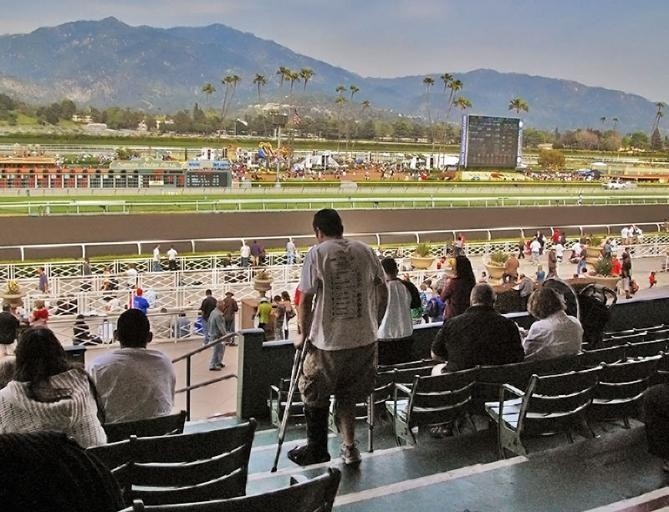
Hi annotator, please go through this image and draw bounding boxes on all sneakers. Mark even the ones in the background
[337,443,364,466]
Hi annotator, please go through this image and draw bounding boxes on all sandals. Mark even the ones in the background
[428,424,453,439]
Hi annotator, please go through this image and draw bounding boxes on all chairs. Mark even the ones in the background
[0,409,341,512]
[267,323,669,458]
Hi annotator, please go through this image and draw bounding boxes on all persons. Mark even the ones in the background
[0,144,638,190]
[57,257,150,346]
[294,210,388,465]
[38,266,48,293]
[202,289,292,371]
[2,326,107,448]
[502,226,657,312]
[2,300,48,356]
[430,283,523,436]
[377,256,422,365]
[523,287,584,360]
[73,314,90,345]
[412,241,487,325]
[153,238,296,338]
[576,193,583,204]
[84,309,176,424]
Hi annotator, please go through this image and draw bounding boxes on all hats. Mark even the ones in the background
[224,290,235,296]
[258,297,270,303]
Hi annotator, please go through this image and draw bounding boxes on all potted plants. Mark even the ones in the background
[409,239,435,268]
[586,257,622,292]
[586,235,604,258]
[484,250,510,279]
[4,281,25,314]
[253,269,273,300]
